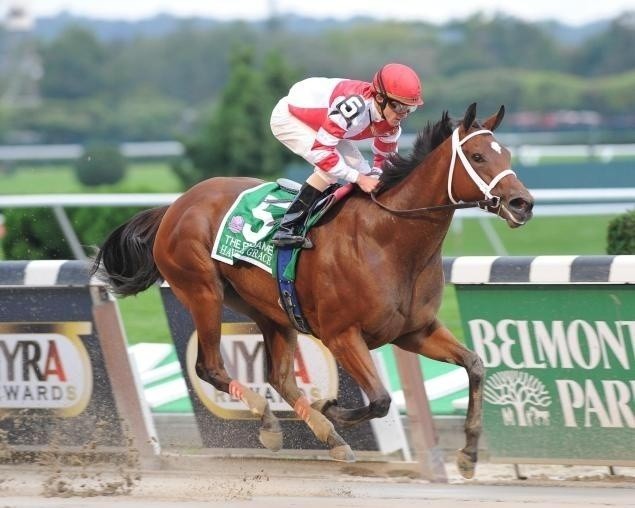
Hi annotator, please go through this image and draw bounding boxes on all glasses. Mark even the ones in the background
[383,93,417,114]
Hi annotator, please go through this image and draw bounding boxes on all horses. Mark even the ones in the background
[88,102,537,478]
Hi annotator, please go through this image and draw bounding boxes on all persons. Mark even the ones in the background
[268,62,424,251]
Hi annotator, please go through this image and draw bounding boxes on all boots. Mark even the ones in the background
[272,180,322,245]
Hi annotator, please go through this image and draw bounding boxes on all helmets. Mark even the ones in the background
[372,63,424,106]
